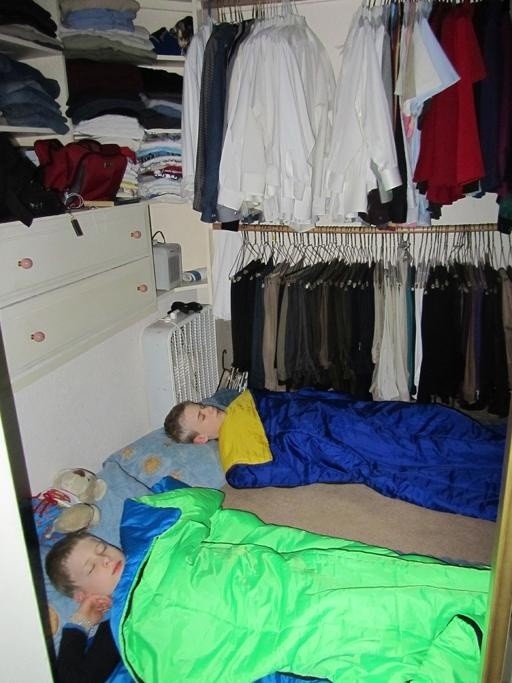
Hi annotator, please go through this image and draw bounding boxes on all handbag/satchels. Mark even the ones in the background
[33,136,128,211]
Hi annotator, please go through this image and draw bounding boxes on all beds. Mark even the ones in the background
[31,395,512,683]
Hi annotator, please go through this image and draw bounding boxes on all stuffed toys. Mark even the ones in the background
[29,466,108,548]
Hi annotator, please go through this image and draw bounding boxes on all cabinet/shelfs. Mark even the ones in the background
[0,1,197,203]
[0,205,212,388]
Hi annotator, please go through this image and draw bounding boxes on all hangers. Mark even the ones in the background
[203,0,305,28]
[358,0,485,17]
[227,223,512,295]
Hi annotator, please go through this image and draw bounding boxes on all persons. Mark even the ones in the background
[45,530,127,683]
[164,399,227,443]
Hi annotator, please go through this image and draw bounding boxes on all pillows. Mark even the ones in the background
[106,387,241,491]
[33,464,157,660]
